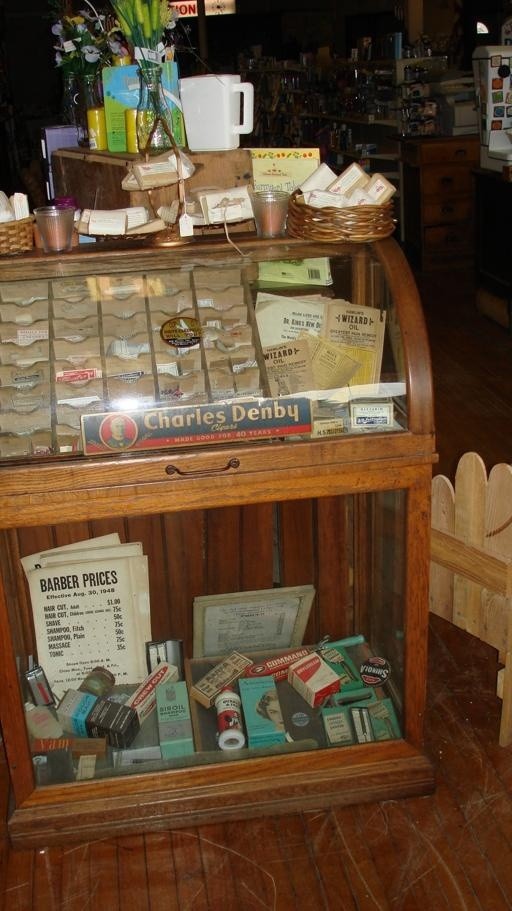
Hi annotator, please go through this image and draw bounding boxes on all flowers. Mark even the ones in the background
[106,1,181,152]
[50,8,125,141]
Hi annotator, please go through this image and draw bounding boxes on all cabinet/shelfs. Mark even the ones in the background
[0,233,442,852]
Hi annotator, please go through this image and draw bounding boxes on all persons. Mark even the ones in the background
[257,688,286,732]
[103,417,134,448]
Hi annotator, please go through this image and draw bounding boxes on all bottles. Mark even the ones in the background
[214,685,246,750]
[25,702,63,740]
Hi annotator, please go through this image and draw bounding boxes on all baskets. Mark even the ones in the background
[0,213,36,256]
[285,186,397,244]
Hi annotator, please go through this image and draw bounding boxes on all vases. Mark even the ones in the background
[60,73,107,151]
[134,68,175,156]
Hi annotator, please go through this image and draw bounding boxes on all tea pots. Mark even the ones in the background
[179,73,255,152]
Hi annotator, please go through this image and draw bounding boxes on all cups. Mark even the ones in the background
[252,190,289,237]
[33,206,75,253]
